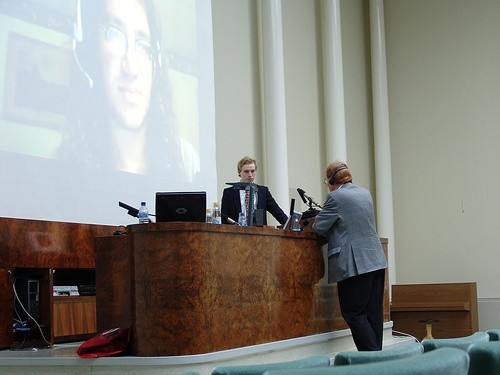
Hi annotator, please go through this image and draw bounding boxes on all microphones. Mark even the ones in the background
[290,199,295,229]
[297,188,307,204]
[221,214,239,225]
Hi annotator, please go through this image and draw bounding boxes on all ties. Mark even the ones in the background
[245,190,249,221]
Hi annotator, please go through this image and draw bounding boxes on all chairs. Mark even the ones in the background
[182,329,500,375]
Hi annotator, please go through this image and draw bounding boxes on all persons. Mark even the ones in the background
[57,0,199,182]
[312,161,388,351]
[221,156,288,226]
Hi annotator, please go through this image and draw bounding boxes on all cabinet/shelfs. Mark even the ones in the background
[0,267,95,349]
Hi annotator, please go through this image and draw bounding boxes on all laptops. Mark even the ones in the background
[283,211,303,230]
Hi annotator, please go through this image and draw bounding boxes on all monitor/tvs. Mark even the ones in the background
[155,192,207,222]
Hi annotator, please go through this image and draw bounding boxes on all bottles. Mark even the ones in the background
[239,213,246,226]
[206,209,212,223]
[139,202,149,224]
[212,203,221,224]
[292,215,300,231]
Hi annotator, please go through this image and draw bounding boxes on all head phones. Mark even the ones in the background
[329,168,346,185]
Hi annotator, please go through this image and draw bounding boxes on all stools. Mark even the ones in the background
[420,319,439,340]
